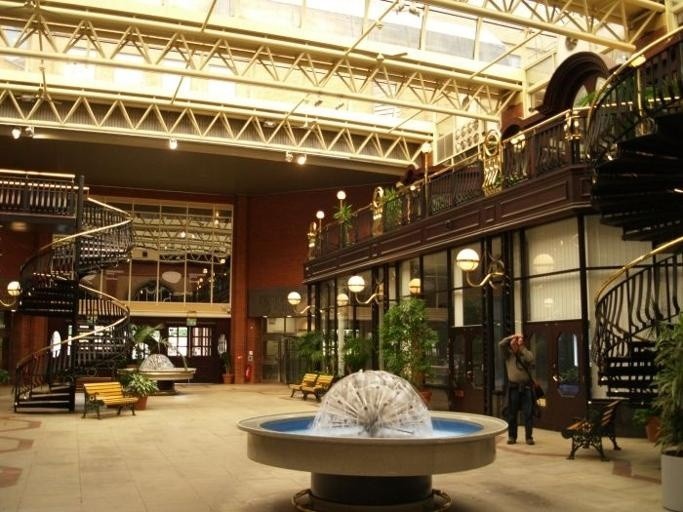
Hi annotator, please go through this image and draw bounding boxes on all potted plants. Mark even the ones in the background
[220,352,235,385]
[376,298,441,411]
[125,373,161,411]
[631,300,683,512]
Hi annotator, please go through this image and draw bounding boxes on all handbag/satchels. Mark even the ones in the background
[533,384,544,400]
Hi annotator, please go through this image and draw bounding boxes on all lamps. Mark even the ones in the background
[287,290,321,317]
[455,248,506,292]
[402,277,422,298]
[346,274,383,307]
[329,292,349,316]
[0,279,22,308]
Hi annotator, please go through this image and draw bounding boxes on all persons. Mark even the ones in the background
[497,332,536,445]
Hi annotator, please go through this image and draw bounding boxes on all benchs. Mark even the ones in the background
[80,378,140,423]
[559,396,626,462]
[289,371,333,403]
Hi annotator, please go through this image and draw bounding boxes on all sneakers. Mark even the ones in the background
[506,438,516,444]
[526,438,534,445]
